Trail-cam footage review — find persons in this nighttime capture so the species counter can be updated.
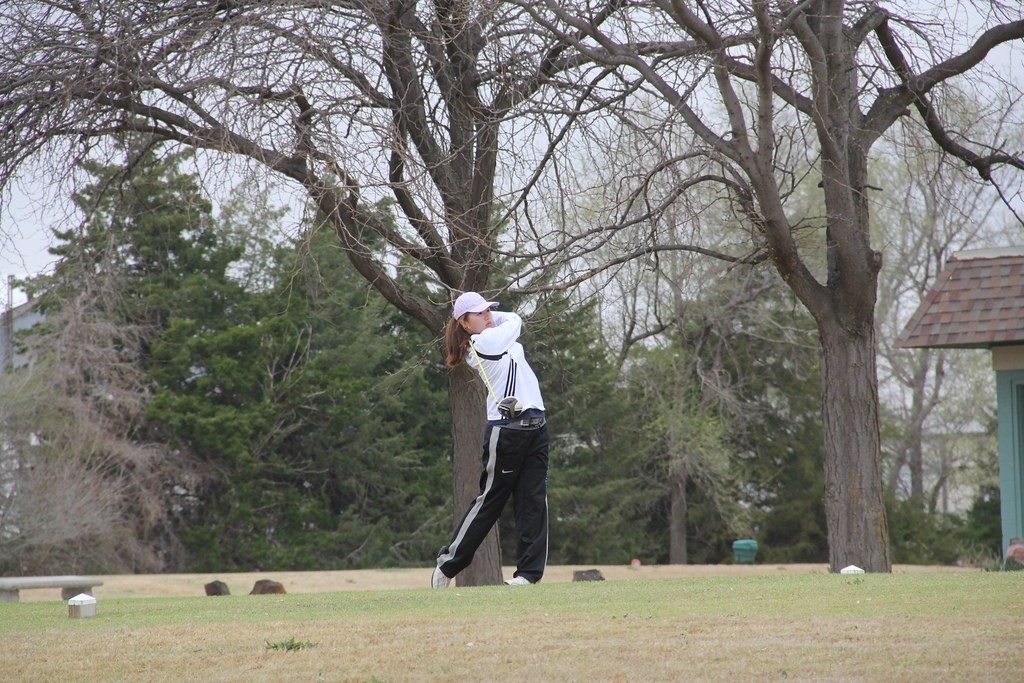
[431,292,550,586]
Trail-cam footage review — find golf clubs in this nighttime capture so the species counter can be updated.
[468,339,517,420]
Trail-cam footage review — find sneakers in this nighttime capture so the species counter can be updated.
[504,576,534,585]
[431,564,452,590]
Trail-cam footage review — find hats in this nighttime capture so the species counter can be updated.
[451,292,499,321]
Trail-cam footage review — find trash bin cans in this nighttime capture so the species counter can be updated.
[731,539,757,563]
